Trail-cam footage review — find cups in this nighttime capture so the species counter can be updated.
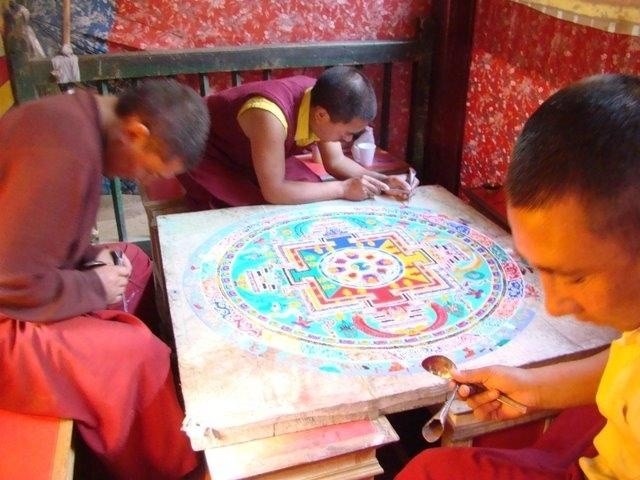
[357,143,377,168]
[311,143,322,163]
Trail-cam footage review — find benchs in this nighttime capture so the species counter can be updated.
[0,410,81,480]
[25,35,415,238]
[465,180,513,231]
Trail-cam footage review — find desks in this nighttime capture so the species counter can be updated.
[152,177,621,479]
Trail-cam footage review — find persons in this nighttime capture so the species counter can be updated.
[174,64,420,211]
[388,73,640,479]
[1,77,209,480]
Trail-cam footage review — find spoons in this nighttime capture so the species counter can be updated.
[422,355,528,414]
